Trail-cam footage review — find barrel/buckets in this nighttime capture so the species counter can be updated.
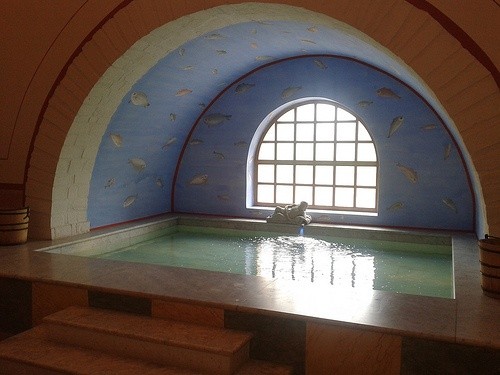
[0,206,31,245]
[478,234,500,293]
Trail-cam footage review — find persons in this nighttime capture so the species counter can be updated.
[270,200,309,219]
